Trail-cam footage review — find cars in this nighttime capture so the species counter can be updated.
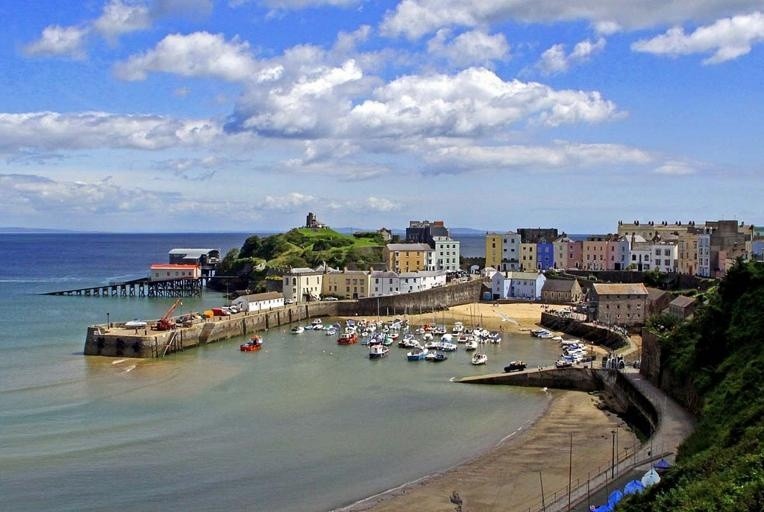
[325,297,338,301]
[504,361,527,373]
[211,305,239,316]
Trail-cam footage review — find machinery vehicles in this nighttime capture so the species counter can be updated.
[150,298,183,331]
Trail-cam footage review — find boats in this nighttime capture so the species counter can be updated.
[125,321,147,329]
[290,317,502,365]
[593,459,673,512]
[528,328,588,363]
[239,335,263,351]
